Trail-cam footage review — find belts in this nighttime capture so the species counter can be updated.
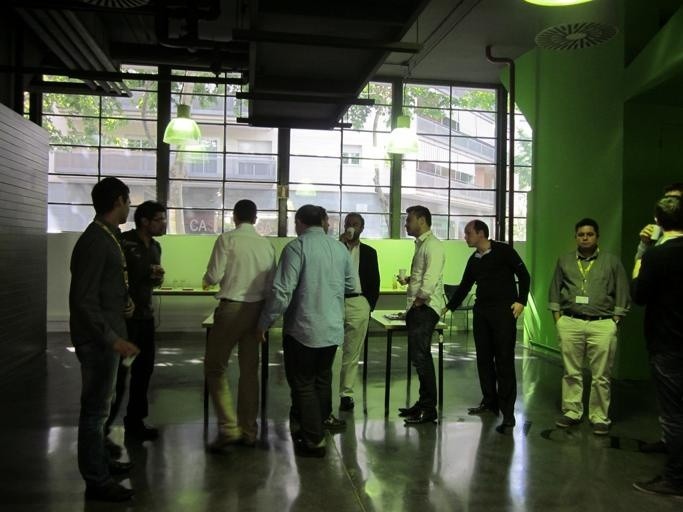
[344,293,363,298]
[563,309,615,323]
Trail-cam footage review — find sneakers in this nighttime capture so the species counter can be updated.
[638,440,664,456]
[556,416,585,429]
[591,422,611,436]
[632,475,682,499]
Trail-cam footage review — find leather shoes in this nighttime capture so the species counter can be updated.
[323,414,346,429]
[402,406,438,426]
[110,459,137,474]
[123,426,159,450]
[497,416,517,430]
[468,401,493,416]
[92,482,138,501]
[399,401,420,415]
[340,396,354,410]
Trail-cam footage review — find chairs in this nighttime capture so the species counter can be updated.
[441,285,476,336]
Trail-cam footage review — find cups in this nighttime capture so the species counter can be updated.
[345,227,355,239]
[200,276,216,292]
[398,268,408,282]
[151,265,161,278]
[649,224,661,241]
[121,346,141,368]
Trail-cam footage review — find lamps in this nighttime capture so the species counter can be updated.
[161,64,201,146]
[385,74,418,154]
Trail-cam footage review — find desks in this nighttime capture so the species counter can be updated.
[197,309,276,438]
[152,286,218,322]
[364,307,448,419]
[379,286,407,298]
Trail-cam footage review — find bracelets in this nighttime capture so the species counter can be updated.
[411,301,422,308]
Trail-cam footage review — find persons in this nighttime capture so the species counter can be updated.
[627,195,683,501]
[632,188,682,455]
[103,199,169,443]
[257,204,353,458]
[314,206,349,431]
[546,217,632,435]
[67,177,142,502]
[396,205,445,426]
[440,220,532,427]
[337,213,379,407]
[203,198,276,457]
